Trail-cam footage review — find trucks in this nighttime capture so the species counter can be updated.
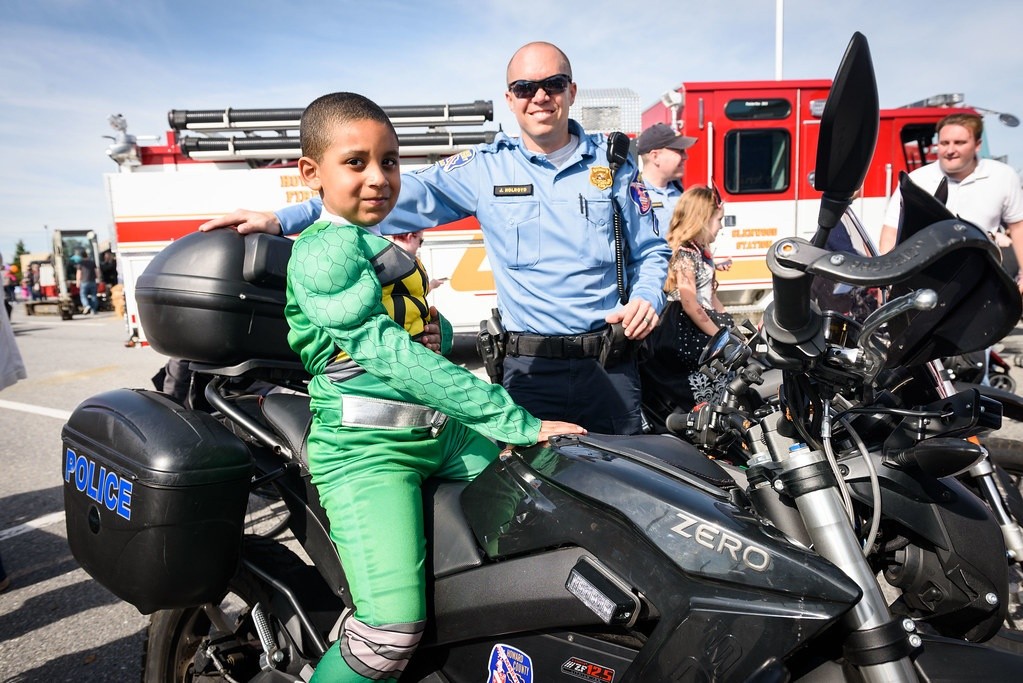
[102,73,1023,367]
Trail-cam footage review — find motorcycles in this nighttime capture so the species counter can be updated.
[59,29,1023,683]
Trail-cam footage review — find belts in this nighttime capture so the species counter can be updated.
[501,332,602,359]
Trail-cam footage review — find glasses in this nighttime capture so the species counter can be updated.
[508,74,572,98]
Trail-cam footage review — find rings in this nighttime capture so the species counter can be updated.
[645,318,650,323]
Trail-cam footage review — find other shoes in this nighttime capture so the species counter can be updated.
[84,306,92,314]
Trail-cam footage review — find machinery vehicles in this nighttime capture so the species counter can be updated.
[21,229,115,317]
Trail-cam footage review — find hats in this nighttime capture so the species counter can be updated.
[638,124,698,155]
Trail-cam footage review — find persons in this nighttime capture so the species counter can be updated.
[392,231,447,296]
[4,264,42,301]
[101,253,117,285]
[285,93,588,683]
[636,123,700,433]
[880,114,1023,393]
[76,251,97,316]
[658,185,732,371]
[201,42,670,432]
